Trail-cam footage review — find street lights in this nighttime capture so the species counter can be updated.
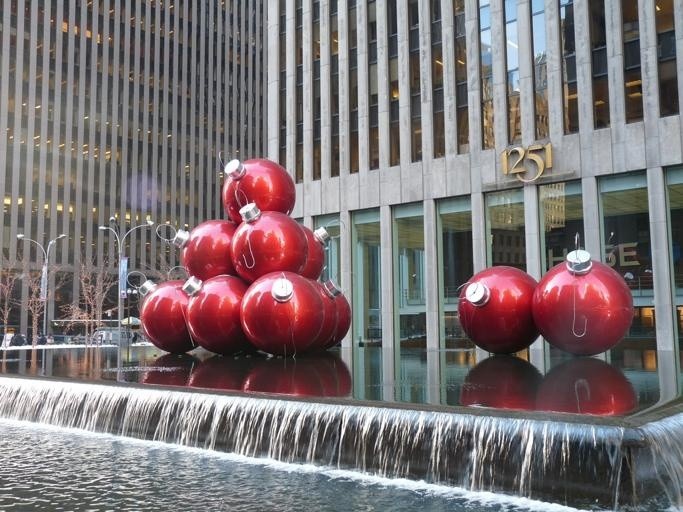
[98,216,154,346]
[16,233,67,336]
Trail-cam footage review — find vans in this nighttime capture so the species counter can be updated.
[94,331,141,345]
